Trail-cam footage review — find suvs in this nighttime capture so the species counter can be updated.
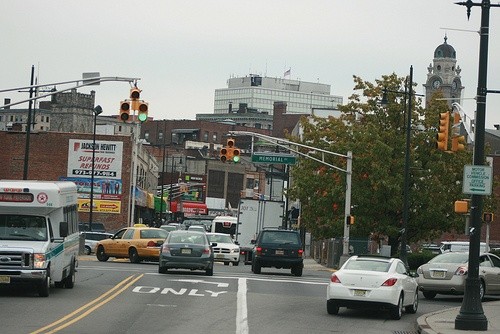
[205,232,241,266]
[80,232,114,255]
[250,227,305,277]
[418,241,490,253]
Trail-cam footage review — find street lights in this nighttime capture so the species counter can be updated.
[120,89,149,121]
[88,105,103,232]
[377,64,424,264]
[6,64,34,182]
[220,139,241,162]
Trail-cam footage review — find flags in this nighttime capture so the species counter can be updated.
[284,70,290,76]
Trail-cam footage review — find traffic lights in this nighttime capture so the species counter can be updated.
[452,112,465,153]
[435,109,450,152]
[347,216,354,224]
[483,213,492,223]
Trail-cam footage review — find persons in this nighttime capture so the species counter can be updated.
[115,181,119,194]
[110,180,115,194]
[106,179,110,194]
[102,180,106,193]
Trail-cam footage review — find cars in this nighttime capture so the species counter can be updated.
[417,251,500,300]
[326,254,419,319]
[95,223,169,263]
[159,230,218,276]
[160,215,237,240]
[79,222,105,233]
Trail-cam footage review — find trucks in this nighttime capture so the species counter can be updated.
[237,197,286,265]
[0,179,80,298]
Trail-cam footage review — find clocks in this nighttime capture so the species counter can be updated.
[433,80,441,88]
[452,81,457,89]
